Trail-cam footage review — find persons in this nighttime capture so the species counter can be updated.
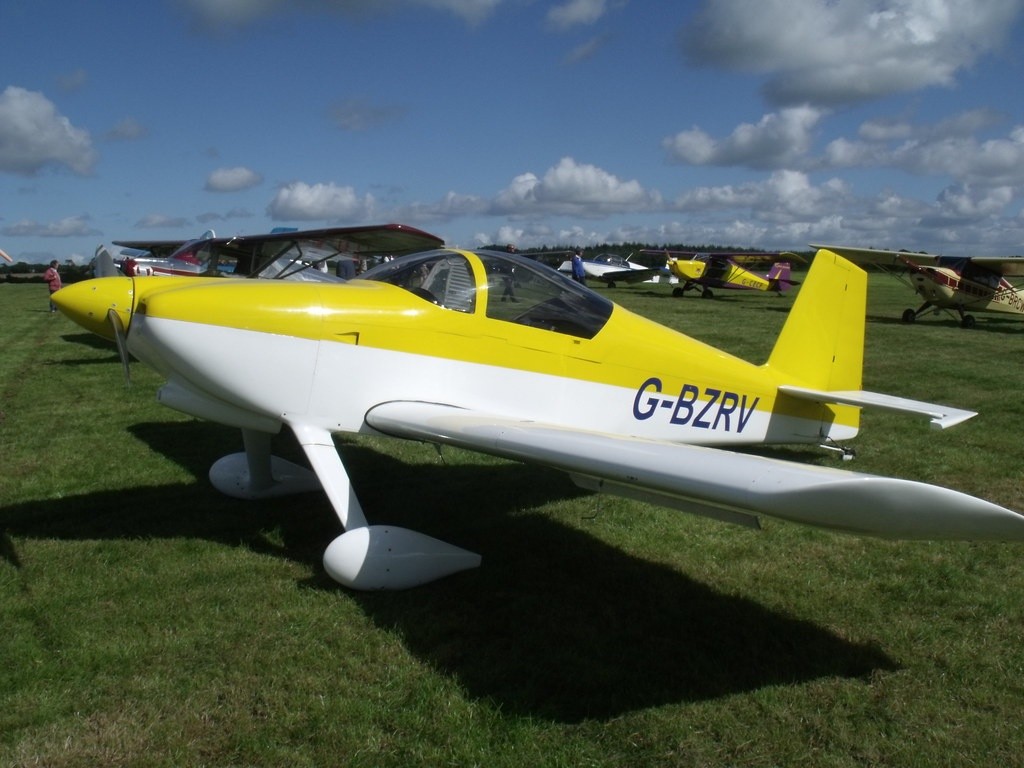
[196,255,238,268]
[571,248,587,288]
[499,243,521,303]
[125,256,130,264]
[43,259,63,313]
[119,258,128,275]
[126,259,136,276]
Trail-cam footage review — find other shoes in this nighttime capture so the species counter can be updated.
[501,299,506,302]
[512,300,520,303]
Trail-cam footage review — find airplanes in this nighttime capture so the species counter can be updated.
[638,244,808,301]
[555,251,678,289]
[38,248,1024,594]
[806,242,1023,330]
[108,223,447,280]
